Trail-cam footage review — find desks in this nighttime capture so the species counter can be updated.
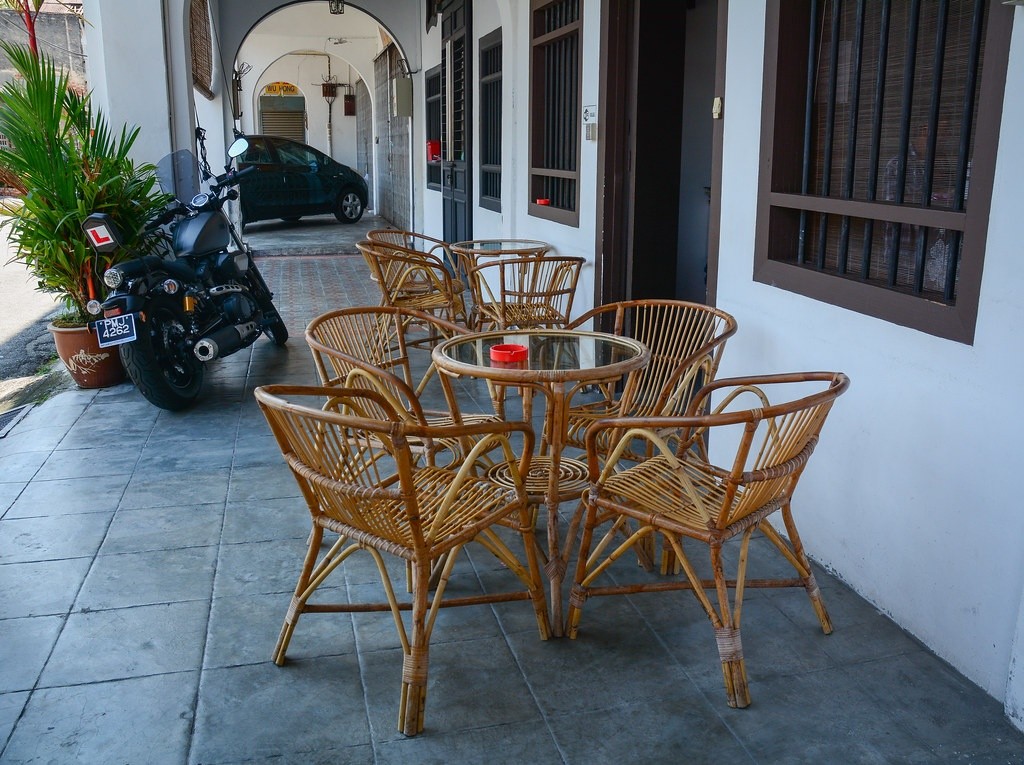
[450,239,551,377]
[431,328,649,638]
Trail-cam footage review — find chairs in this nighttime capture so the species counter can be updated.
[253,229,850,734]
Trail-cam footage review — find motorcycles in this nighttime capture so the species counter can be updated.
[85,137,289,413]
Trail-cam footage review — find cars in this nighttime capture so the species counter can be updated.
[235,134,369,228]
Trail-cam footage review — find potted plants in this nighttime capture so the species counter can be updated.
[0,0,175,388]
